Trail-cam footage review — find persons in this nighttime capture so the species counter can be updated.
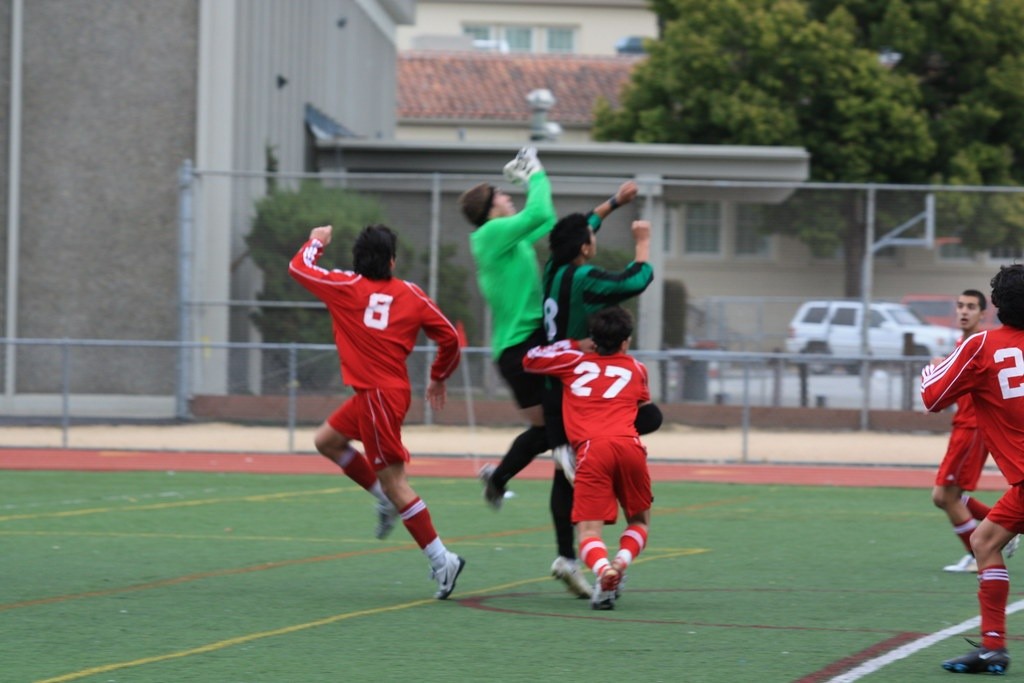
[458,145,562,508]
[288,223,466,601]
[523,305,652,612]
[921,264,1024,673]
[541,180,663,597]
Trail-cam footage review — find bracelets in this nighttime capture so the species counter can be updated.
[609,196,619,210]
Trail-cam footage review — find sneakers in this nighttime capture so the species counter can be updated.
[477,462,507,511]
[610,556,627,586]
[940,647,1010,675]
[549,555,595,600]
[1003,533,1020,559]
[943,553,981,573]
[430,550,466,600]
[590,563,620,611]
[373,500,399,540]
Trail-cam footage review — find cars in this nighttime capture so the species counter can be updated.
[786,300,964,375]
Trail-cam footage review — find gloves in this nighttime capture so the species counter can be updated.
[512,146,543,179]
[502,160,529,184]
